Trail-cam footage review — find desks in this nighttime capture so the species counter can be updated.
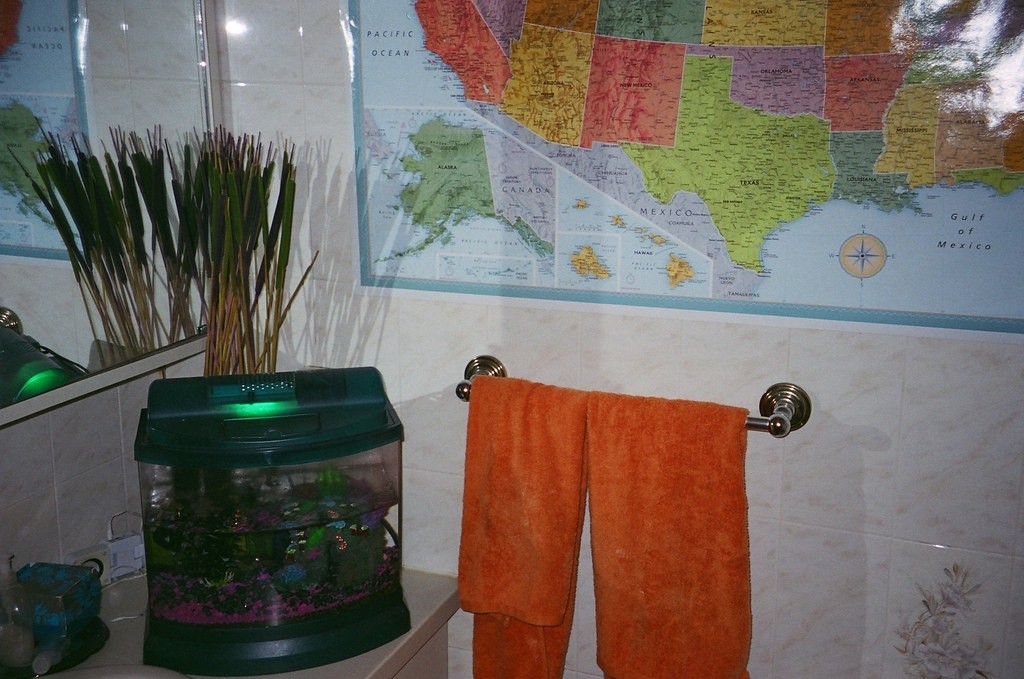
[34,563,462,679]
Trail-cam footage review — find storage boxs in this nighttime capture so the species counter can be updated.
[133,366,411,676]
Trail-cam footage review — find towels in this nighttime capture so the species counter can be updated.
[455,373,589,679]
[585,387,757,679]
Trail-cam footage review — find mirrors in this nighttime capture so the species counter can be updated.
[0,0,221,427]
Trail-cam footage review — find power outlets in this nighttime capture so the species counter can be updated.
[64,545,111,589]
[100,532,141,581]
